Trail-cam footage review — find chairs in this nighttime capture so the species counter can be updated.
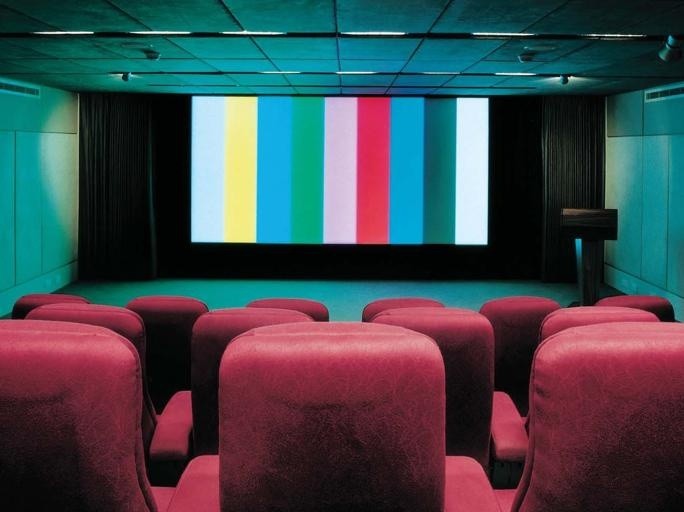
[21,301,163,462]
[478,294,563,415]
[595,294,678,320]
[445,320,682,509]
[359,296,447,323]
[122,296,210,394]
[2,317,177,510]
[163,317,446,512]
[487,304,664,492]
[369,306,497,471]
[244,296,334,327]
[145,307,317,484]
[9,292,87,316]
